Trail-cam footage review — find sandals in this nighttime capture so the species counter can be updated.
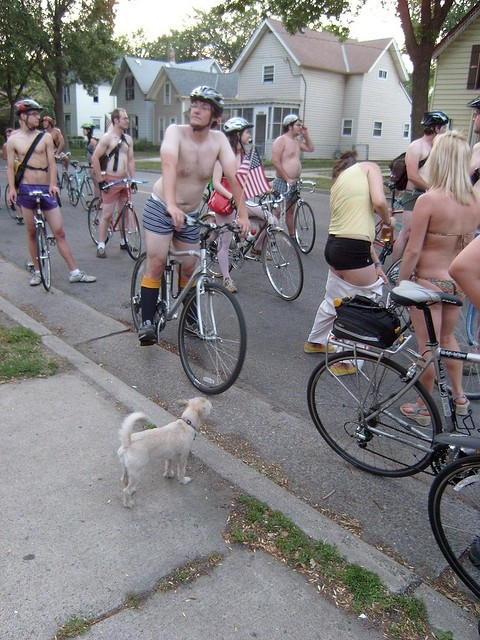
[400,404,432,426]
[452,395,470,414]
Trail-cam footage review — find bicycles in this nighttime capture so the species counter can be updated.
[200,190,304,301]
[277,179,316,254]
[4,182,23,220]
[129,210,248,395]
[67,159,100,211]
[88,177,150,260]
[54,150,75,204]
[373,180,405,264]
[436,297,480,400]
[425,436,477,598]
[11,189,56,292]
[306,279,480,479]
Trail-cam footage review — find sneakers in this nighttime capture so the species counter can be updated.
[251,249,274,260]
[70,272,96,282]
[97,247,106,260]
[304,342,337,354]
[330,363,358,376]
[184,319,212,340]
[30,272,42,285]
[120,243,139,252]
[138,320,158,346]
[223,278,237,294]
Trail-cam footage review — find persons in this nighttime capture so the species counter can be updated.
[91,108,139,259]
[212,116,279,294]
[0,127,13,158]
[466,94,480,206]
[269,114,315,248]
[392,111,449,270]
[448,235,480,316]
[396,128,480,428]
[139,86,250,345]
[304,151,396,376]
[7,100,98,285]
[42,115,69,182]
[81,123,100,211]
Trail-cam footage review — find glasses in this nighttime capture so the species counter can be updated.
[25,112,41,116]
[192,104,213,111]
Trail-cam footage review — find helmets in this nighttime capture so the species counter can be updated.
[80,122,95,129]
[42,116,53,123]
[13,99,43,115]
[465,95,480,108]
[190,86,226,111]
[223,117,253,132]
[420,111,449,125]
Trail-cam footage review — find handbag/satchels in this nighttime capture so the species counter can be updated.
[208,169,238,215]
[96,134,126,171]
[388,153,429,191]
[13,130,46,187]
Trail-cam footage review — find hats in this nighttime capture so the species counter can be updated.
[283,115,299,126]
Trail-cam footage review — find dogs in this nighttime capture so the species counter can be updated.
[118,396,214,509]
